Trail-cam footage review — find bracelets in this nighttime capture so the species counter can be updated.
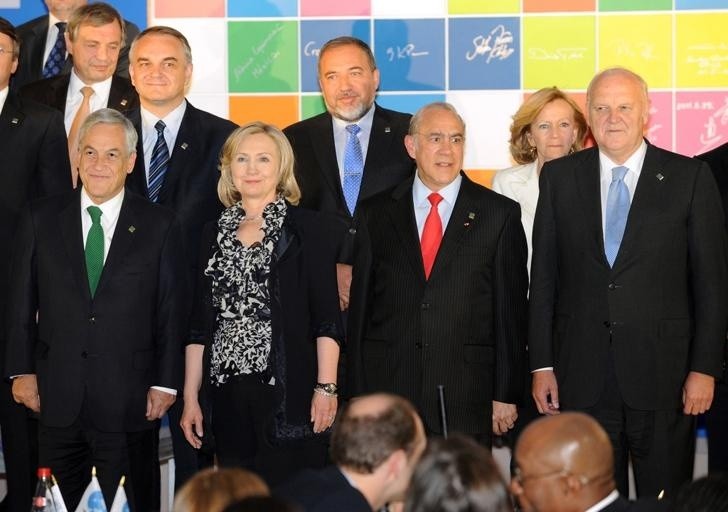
[314,382,340,398]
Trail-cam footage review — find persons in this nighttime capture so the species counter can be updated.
[271,393,429,511]
[19,3,140,192]
[118,26,241,512]
[506,408,681,512]
[526,66,723,503]
[1,16,74,512]
[690,144,728,483]
[490,84,591,304]
[8,107,187,512]
[285,36,419,402]
[170,466,271,511]
[179,122,345,490]
[346,102,530,460]
[401,431,515,512]
[7,1,143,92]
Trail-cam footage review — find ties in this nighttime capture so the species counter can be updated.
[84,206,104,300]
[342,124,364,218]
[420,192,444,281]
[42,22,66,80]
[147,120,169,203]
[67,87,95,190]
[604,166,631,270]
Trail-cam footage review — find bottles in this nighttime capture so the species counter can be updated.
[31,467,58,512]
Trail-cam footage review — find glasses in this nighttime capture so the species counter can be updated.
[0,48,13,56]
[515,466,570,485]
